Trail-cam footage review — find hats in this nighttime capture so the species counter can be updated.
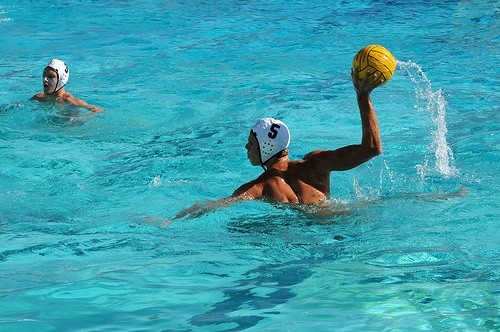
[44,59,69,95]
[250,116,290,163]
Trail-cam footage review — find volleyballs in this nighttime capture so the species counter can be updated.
[350,43,396,87]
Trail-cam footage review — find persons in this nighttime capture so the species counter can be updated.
[168,66,383,222]
[0,58,105,122]
